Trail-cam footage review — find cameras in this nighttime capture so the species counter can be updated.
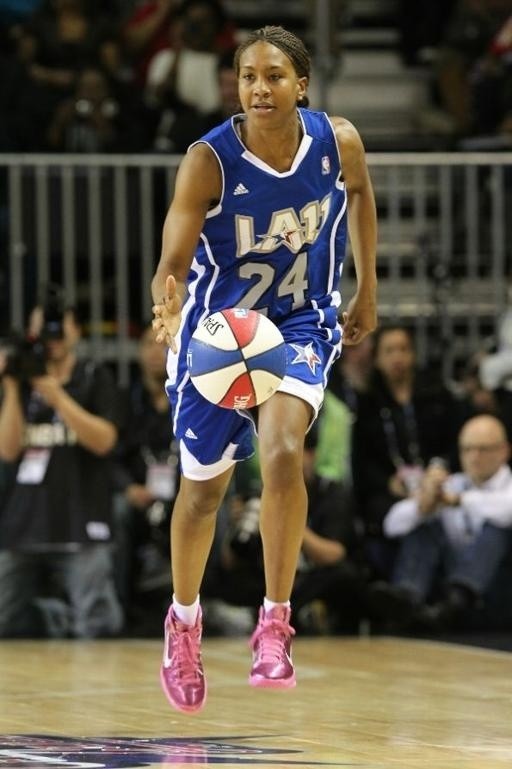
[6,335,47,381]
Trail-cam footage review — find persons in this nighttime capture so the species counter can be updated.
[0,1,512,340]
[151,26,377,710]
[1,304,512,639]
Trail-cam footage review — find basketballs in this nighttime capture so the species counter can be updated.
[188,309,287,410]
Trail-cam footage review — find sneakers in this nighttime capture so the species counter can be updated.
[248,603,297,690]
[158,603,207,715]
